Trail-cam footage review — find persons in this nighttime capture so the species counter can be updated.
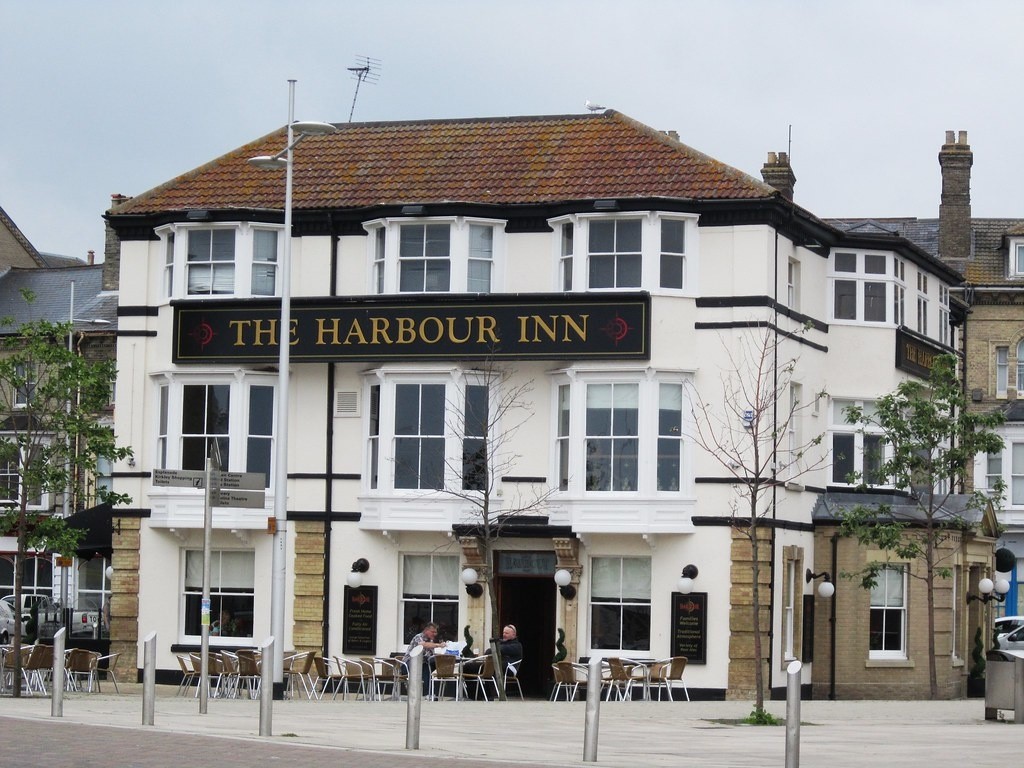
[103,596,112,636]
[400,622,447,701]
[209,609,234,636]
[482,624,523,701]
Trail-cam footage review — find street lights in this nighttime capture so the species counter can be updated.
[59,279,110,629]
[249,78,337,699]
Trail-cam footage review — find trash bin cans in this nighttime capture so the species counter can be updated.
[983,649,1024,724]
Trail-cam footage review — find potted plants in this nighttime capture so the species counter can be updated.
[967,627,985,697]
[543,628,573,701]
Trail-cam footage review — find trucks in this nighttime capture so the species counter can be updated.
[1,593,103,643]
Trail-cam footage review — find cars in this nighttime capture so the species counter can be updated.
[987,615,1024,661]
[0,599,30,657]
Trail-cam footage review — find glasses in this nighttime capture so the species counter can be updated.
[507,625,515,633]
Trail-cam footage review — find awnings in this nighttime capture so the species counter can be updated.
[63,501,111,561]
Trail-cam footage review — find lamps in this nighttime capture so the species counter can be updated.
[553,570,576,599]
[593,200,620,209]
[679,565,698,593]
[461,568,483,598]
[346,558,369,587]
[794,238,823,247]
[186,211,212,219]
[401,206,427,214]
[989,579,1010,602]
[806,568,834,597]
[967,578,993,605]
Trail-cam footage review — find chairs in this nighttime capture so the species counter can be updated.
[0,643,122,696]
[177,650,523,704]
[549,656,692,702]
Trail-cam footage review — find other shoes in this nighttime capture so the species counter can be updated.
[487,696,494,701]
[425,694,438,701]
